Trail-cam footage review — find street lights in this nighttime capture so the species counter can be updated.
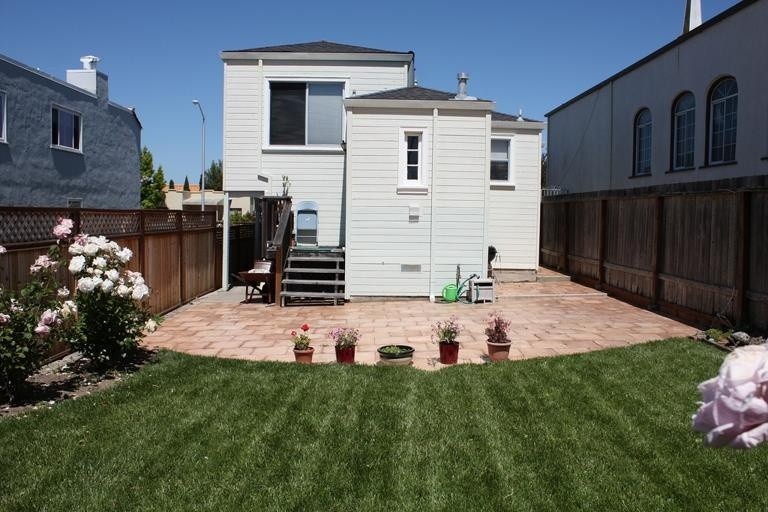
[192,99,207,220]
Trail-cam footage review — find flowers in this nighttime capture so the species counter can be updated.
[329,326,360,348]
[425,313,464,344]
[290,324,311,350]
[482,307,513,344]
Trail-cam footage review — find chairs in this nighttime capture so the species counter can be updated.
[240,258,274,303]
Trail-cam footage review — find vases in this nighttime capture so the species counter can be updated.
[292,346,314,365]
[487,340,512,364]
[437,342,460,365]
[335,346,355,366]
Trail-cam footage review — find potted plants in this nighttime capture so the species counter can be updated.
[376,342,416,368]
